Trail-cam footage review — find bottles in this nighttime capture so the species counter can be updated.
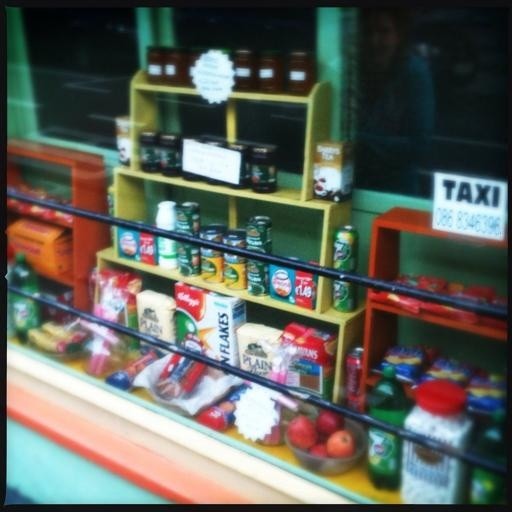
[138,128,281,194]
[145,44,316,96]
[400,378,475,506]
[467,408,508,504]
[365,365,411,491]
[8,252,41,343]
[155,200,180,269]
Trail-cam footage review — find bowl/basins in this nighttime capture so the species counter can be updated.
[284,411,366,476]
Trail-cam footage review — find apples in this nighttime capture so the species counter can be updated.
[286,409,357,458]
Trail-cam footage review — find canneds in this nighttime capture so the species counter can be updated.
[332,279,357,312]
[144,45,311,97]
[333,225,358,271]
[177,201,271,297]
[343,347,364,396]
[139,130,279,194]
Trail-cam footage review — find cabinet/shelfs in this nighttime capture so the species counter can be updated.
[8,136,110,322]
[93,71,369,409]
[356,205,512,417]
